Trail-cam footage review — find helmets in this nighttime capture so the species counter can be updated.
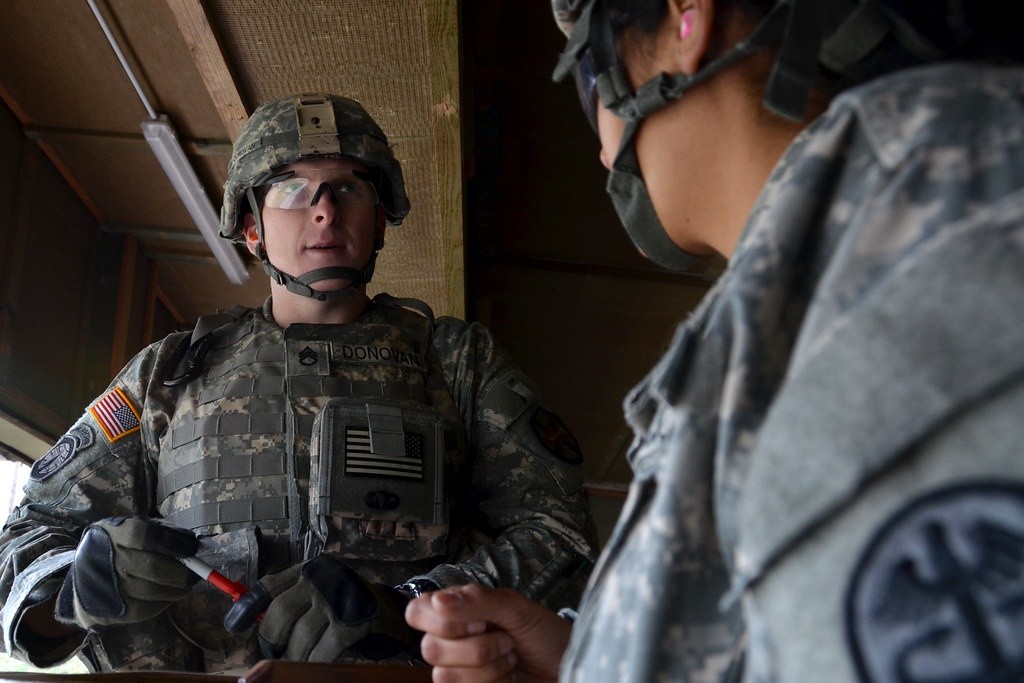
[220,94,411,237]
[551,0,952,275]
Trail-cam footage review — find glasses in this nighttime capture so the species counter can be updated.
[266,168,380,210]
[574,48,602,138]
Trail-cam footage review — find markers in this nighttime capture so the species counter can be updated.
[177,556,267,621]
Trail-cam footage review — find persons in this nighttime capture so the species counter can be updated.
[0,91,599,683]
[405,0,1024,683]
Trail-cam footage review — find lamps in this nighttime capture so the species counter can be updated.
[141,113,251,287]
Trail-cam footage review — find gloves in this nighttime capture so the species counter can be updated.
[55,514,202,628]
[224,558,408,661]
